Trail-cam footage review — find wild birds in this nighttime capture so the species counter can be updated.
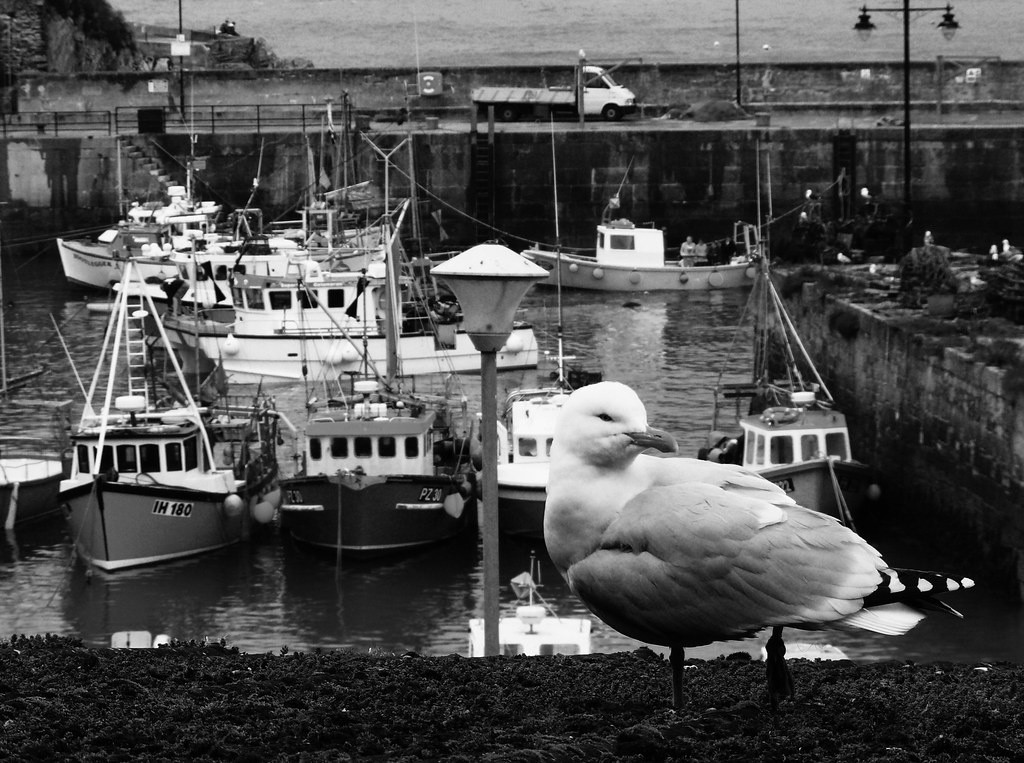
[989,239,1024,267]
[762,44,771,52]
[799,211,808,223]
[860,187,875,206]
[837,252,852,266]
[578,48,588,62]
[804,188,824,204]
[868,262,880,275]
[714,39,719,48]
[543,380,980,716]
[924,229,935,248]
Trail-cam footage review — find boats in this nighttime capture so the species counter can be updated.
[519,218,759,293]
[467,372,584,546]
[0,450,70,533]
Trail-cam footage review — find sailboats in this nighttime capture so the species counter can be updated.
[57,237,279,574]
[694,153,882,526]
[272,204,486,556]
[0,1,606,389]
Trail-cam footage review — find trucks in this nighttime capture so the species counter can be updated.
[469,66,636,123]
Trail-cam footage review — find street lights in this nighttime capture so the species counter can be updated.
[853,0,962,256]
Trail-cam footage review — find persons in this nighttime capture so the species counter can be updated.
[680,236,737,265]
[220,20,240,36]
